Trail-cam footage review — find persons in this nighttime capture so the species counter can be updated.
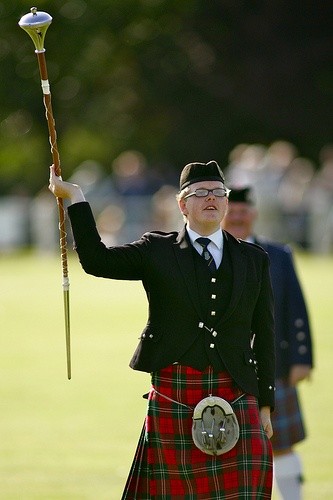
[222,184,316,500]
[48,161,277,500]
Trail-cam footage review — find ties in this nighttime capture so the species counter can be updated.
[195,238,216,274]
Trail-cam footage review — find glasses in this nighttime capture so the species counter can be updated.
[183,188,227,200]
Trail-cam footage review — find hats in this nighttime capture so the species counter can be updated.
[180,160,225,190]
[229,186,255,204]
